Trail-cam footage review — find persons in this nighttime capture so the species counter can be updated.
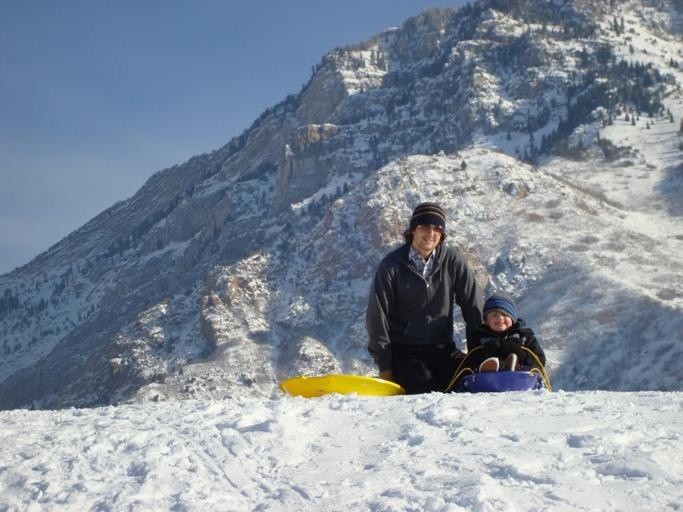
[478,293,545,373]
[365,202,486,395]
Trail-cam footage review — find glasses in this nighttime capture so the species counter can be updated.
[417,223,443,233]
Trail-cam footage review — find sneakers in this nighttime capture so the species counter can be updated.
[503,353,521,371]
[479,357,499,372]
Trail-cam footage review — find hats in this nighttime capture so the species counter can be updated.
[483,291,518,324]
[410,203,446,235]
